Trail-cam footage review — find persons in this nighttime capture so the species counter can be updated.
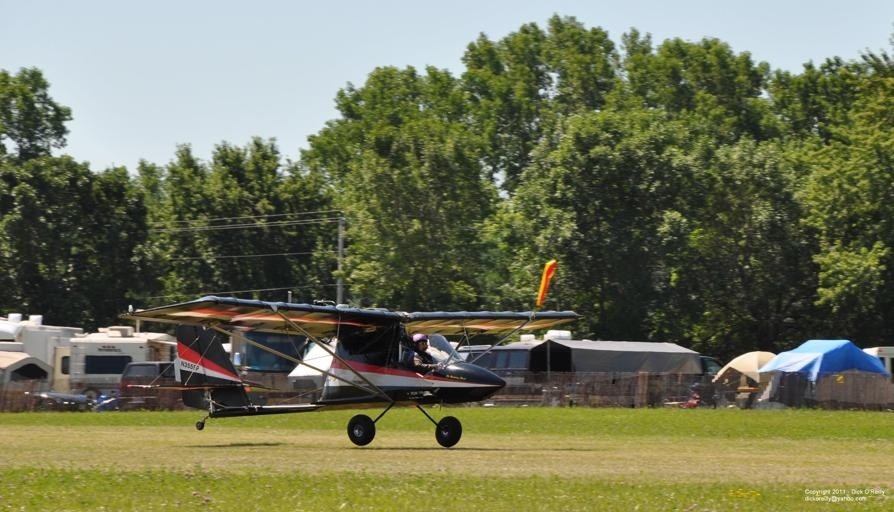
[410,334,444,369]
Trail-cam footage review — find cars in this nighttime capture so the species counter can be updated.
[243,380,282,406]
[32,390,118,412]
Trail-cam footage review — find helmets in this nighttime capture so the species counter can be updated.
[413,334,428,343]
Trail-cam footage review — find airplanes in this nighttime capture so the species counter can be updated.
[119,295,584,448]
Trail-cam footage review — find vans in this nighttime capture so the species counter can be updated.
[119,360,183,412]
